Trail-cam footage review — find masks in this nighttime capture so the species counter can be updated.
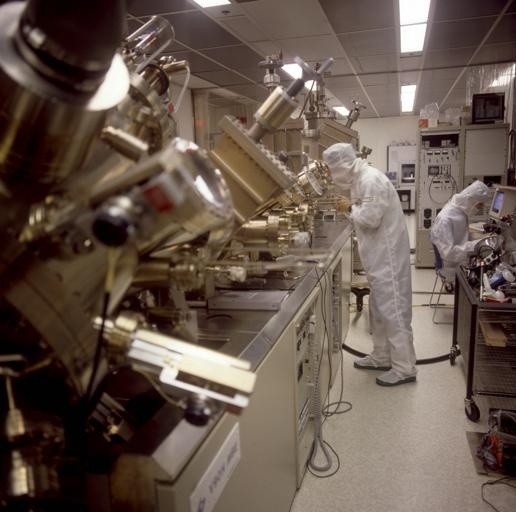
[471,206,480,216]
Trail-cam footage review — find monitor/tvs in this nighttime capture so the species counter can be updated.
[489,186,516,224]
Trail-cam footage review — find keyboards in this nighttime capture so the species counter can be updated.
[469,222,486,231]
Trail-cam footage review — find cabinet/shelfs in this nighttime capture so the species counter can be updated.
[450,262,516,422]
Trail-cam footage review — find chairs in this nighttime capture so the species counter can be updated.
[427,231,458,325]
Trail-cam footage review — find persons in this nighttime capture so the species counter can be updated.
[429,180,491,284]
[322,142,417,386]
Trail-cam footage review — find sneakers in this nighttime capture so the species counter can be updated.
[375,367,417,387]
[353,353,393,371]
